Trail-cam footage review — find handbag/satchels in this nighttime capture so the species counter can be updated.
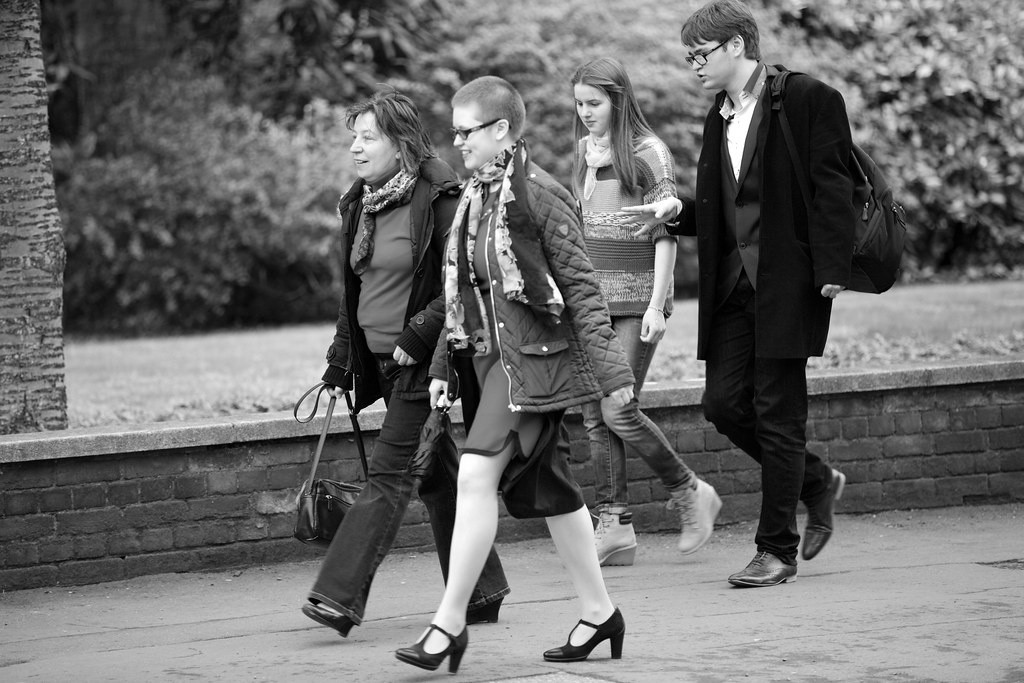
[293,478,364,552]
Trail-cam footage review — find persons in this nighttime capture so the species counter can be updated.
[614,0,857,586]
[570,59,723,566]
[302,86,512,638]
[394,76,637,674]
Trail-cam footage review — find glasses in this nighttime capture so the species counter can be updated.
[448,117,512,141]
[685,35,735,68]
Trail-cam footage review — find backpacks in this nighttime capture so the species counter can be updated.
[772,69,906,295]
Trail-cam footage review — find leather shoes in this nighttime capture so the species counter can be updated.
[800,469,846,561]
[727,551,798,587]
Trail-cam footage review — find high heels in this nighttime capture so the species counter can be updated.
[466,596,504,625]
[589,506,638,568]
[301,604,354,638]
[543,606,626,663]
[666,479,723,555]
[395,622,468,674]
